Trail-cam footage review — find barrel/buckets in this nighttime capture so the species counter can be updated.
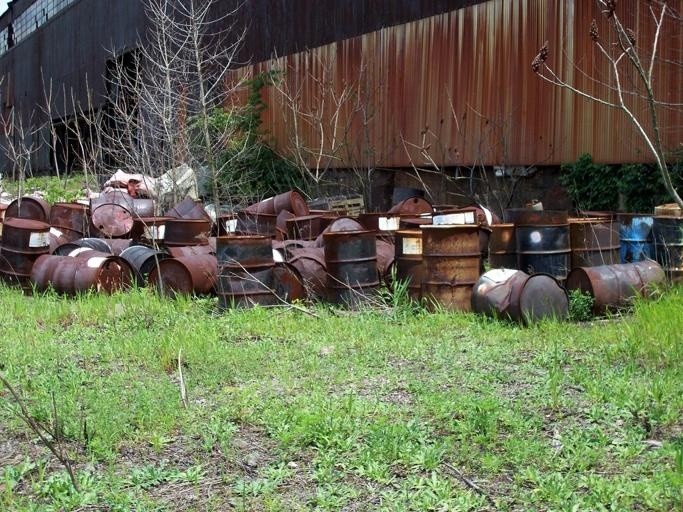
[0,167,683,328]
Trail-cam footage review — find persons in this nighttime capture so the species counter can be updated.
[127,179,150,199]
